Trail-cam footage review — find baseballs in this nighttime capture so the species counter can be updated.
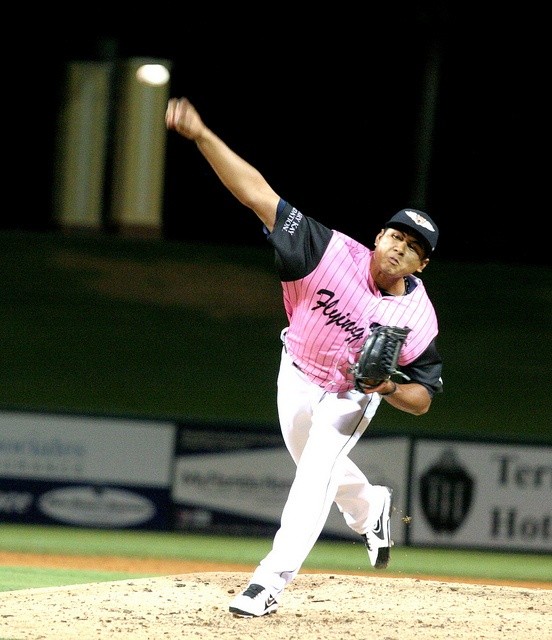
[136,64,169,86]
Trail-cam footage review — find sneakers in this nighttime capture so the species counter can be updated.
[228,584,278,617]
[360,485,394,569]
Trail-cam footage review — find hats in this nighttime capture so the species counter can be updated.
[385,208,440,252]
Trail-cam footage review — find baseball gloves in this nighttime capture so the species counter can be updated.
[348,322,411,394]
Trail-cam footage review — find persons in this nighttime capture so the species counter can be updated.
[165,97,444,617]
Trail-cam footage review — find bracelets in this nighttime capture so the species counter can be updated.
[379,382,397,396]
